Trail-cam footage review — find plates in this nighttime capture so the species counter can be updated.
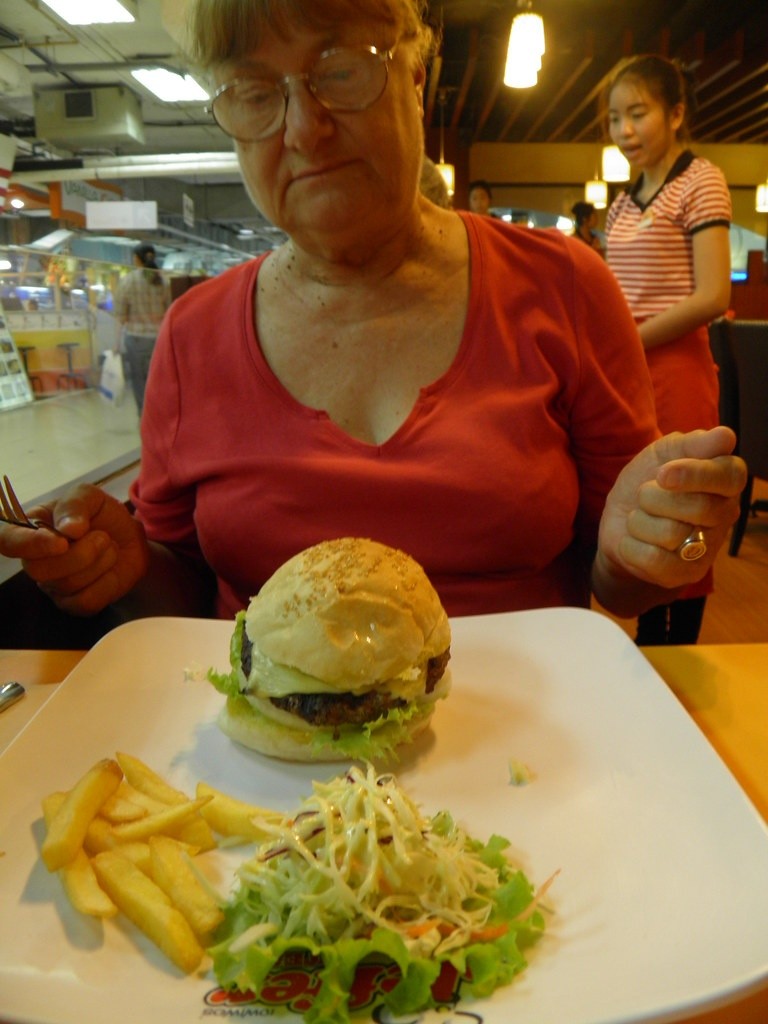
[1,608,768,1023]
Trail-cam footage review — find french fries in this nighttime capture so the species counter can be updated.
[40,752,292,975]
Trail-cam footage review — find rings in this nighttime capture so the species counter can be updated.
[671,526,707,560]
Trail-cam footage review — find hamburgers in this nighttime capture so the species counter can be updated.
[205,535,452,766]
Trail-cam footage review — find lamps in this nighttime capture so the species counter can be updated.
[584,142,635,211]
[432,86,456,197]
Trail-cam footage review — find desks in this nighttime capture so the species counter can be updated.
[1,648,768,1024]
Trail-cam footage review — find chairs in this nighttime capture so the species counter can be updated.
[709,314,767,556]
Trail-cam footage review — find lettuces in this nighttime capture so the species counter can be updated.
[209,809,545,1024]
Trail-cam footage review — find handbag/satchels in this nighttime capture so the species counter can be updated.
[97,351,126,406]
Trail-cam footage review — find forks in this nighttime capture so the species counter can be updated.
[2,471,79,545]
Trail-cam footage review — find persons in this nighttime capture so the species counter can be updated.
[585,54,732,646]
[1,0,746,626]
[566,200,604,259]
[466,179,500,220]
[110,239,171,423]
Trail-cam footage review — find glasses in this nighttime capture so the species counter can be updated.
[204,30,405,140]
